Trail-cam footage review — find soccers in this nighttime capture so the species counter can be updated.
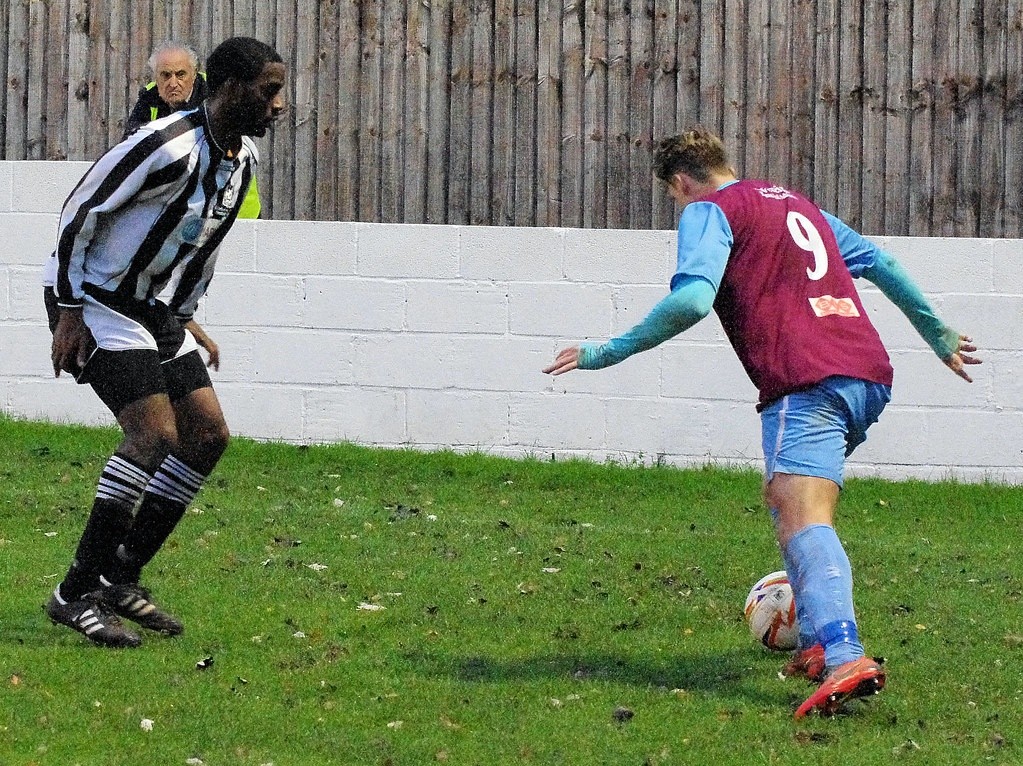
[743,570,800,650]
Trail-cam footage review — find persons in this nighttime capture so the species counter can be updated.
[121,42,262,220]
[44,38,289,648]
[542,129,984,717]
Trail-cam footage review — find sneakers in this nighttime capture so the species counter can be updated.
[795,656,887,719]
[787,642,828,683]
[46,580,143,649]
[98,574,184,636]
